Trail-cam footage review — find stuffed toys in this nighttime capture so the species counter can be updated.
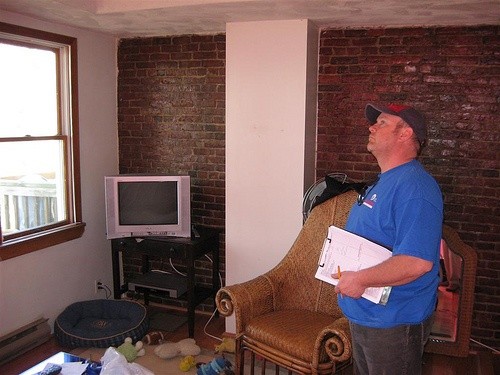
[214,337,236,356]
[114,338,146,362]
[154,337,201,359]
[179,355,195,371]
[196,354,237,375]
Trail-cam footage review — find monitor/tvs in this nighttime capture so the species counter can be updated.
[103,174,194,240]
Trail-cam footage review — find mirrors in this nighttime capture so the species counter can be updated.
[423,223,478,358]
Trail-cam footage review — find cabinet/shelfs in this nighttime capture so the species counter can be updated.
[104,228,225,340]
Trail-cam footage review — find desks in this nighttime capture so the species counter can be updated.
[16,352,101,375]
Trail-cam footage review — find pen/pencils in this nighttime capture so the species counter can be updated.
[338,266,343,300]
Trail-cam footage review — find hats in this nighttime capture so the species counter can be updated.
[365,102,429,149]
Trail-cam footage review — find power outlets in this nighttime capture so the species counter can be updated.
[94,278,104,295]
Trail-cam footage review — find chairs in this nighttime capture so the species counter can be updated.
[214,189,362,375]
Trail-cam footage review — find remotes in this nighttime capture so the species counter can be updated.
[193,230,200,237]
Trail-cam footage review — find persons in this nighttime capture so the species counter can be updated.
[330,103,445,375]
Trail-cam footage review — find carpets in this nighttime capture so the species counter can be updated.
[66,338,293,375]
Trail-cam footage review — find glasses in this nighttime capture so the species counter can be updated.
[356,173,380,206]
